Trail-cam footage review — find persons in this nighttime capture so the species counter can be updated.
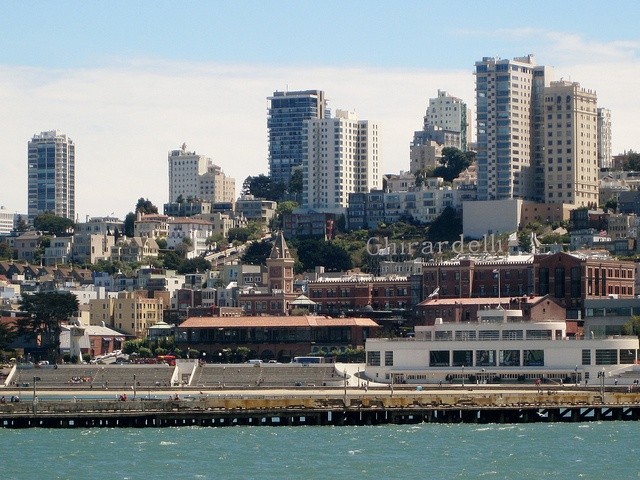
[0,395,20,403]
[67,376,91,384]
[169,395,181,401]
